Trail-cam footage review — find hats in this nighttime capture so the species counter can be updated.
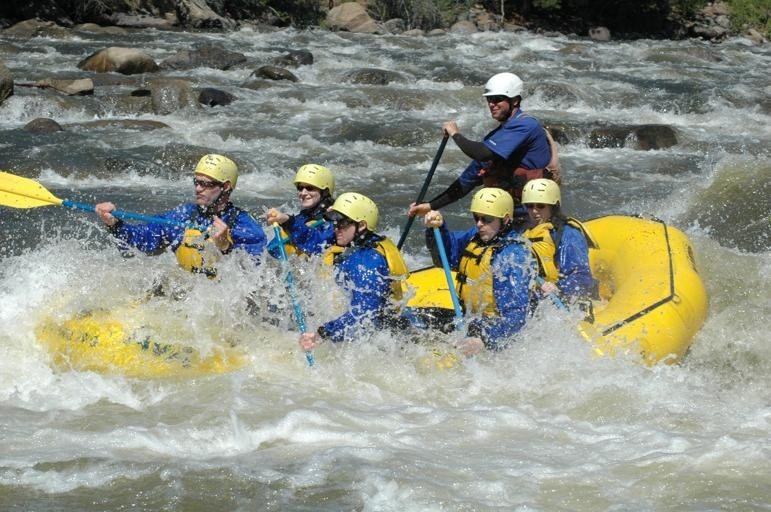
[322,211,347,222]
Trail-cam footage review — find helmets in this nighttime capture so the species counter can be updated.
[482,73,523,98]
[330,192,379,232]
[294,164,334,196]
[521,178,562,208]
[470,188,514,221]
[195,153,239,190]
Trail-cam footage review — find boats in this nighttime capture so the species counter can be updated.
[32,213,709,393]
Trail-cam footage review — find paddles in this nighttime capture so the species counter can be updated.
[0,169,214,235]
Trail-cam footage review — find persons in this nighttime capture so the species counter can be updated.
[519,177,601,325]
[266,191,410,354]
[96,153,268,280]
[424,187,538,359]
[408,72,561,233]
[266,165,334,260]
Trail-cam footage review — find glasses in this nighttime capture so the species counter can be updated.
[474,214,497,223]
[525,204,545,210]
[487,96,504,103]
[297,185,315,191]
[194,178,218,188]
[333,219,352,229]
